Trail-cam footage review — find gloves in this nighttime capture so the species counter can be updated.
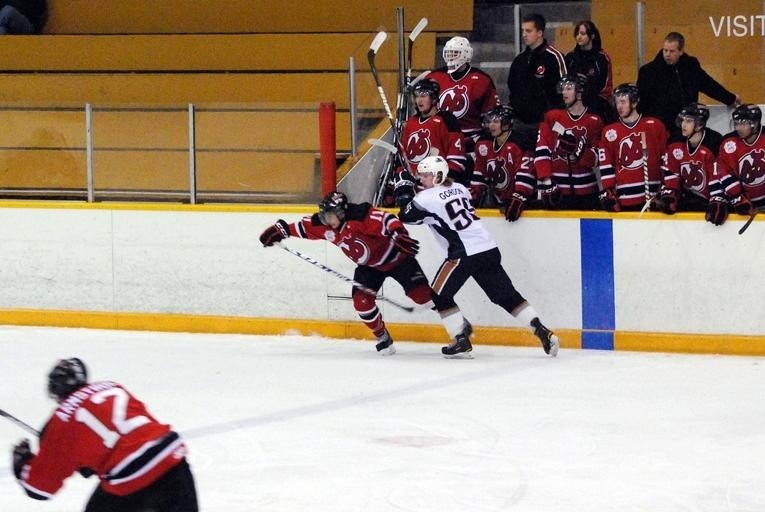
[14,441,31,478]
[500,192,528,222]
[388,230,419,257]
[468,184,487,208]
[556,134,584,160]
[392,169,422,199]
[729,192,760,215]
[259,220,290,247]
[705,193,730,226]
[650,184,679,215]
[541,183,564,210]
[597,188,621,212]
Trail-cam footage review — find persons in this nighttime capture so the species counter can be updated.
[717,102,765,215]
[634,33,742,153]
[12,356,198,511]
[393,155,554,356]
[394,78,475,208]
[656,102,728,225]
[534,73,604,210]
[599,82,668,213]
[507,13,568,147]
[469,105,536,222]
[402,35,500,166]
[564,22,616,123]
[260,190,472,351]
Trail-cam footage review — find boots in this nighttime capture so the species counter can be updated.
[375,328,393,351]
[442,328,472,354]
[531,317,553,354]
[462,315,472,336]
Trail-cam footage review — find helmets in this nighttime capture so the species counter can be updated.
[443,36,474,74]
[417,156,449,177]
[730,104,762,131]
[49,358,86,401]
[556,72,587,94]
[609,83,639,107]
[318,191,347,226]
[412,78,440,100]
[481,105,513,132]
[675,103,710,128]
